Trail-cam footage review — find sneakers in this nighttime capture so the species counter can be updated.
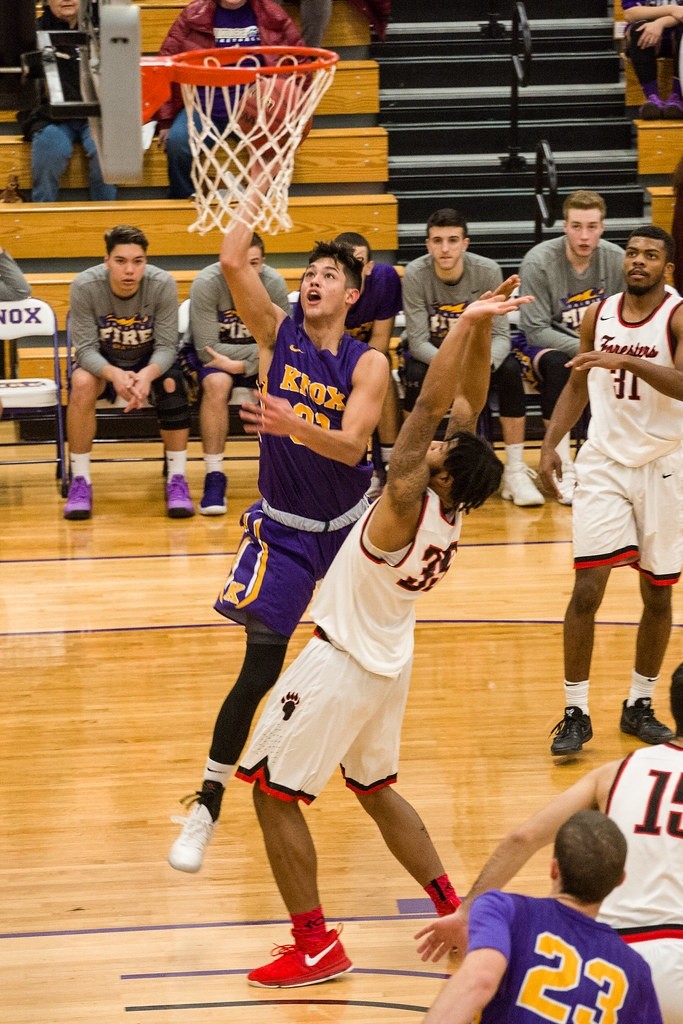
[620,697,674,743]
[63,476,92,520]
[502,462,545,506]
[167,804,218,873]
[246,922,353,989]
[548,706,593,755]
[551,462,575,504]
[165,474,194,517]
[663,93,683,119]
[198,472,227,515]
[639,94,661,119]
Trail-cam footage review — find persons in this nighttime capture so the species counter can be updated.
[155,0,313,201]
[534,227,683,759]
[233,274,534,991]
[335,232,399,498]
[400,211,547,506]
[420,809,666,1024]
[275,0,332,60]
[621,0,683,120]
[21,0,118,203]
[414,662,683,1024]
[516,191,632,505]
[170,154,388,876]
[119,234,291,543]
[0,245,31,302]
[62,227,196,518]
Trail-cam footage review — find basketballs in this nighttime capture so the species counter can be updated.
[236,78,314,159]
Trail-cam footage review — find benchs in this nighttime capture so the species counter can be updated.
[0,0,400,411]
[613,0,683,290]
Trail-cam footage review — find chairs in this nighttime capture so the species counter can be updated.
[0,297,68,498]
